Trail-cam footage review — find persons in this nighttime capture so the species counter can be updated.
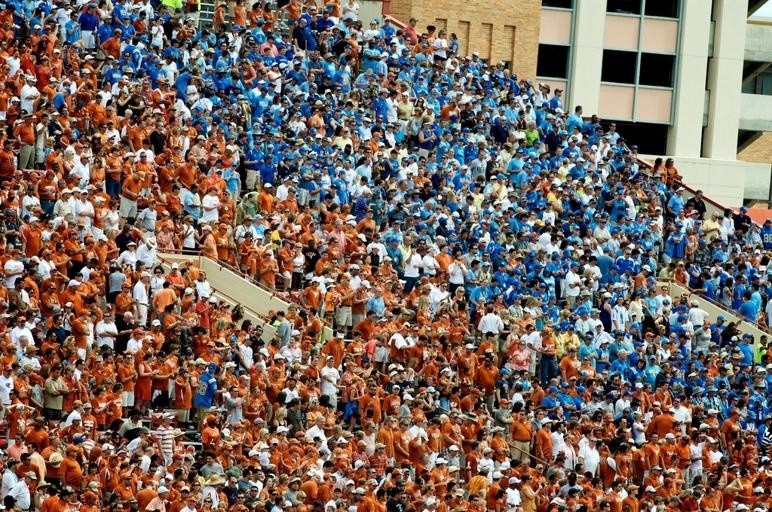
[0,0,770,512]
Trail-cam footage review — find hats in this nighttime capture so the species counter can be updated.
[2,5,771,510]
